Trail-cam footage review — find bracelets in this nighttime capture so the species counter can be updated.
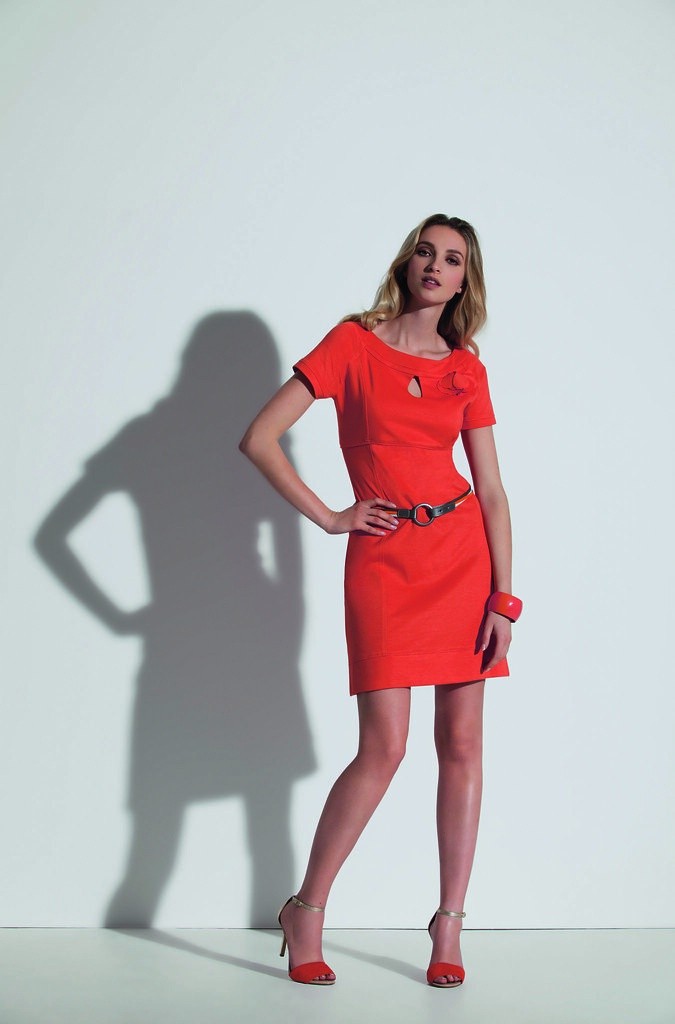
[486,592,523,622]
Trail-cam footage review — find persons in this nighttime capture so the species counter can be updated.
[239,214,513,987]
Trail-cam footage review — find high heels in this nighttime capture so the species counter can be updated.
[278,895,336,986]
[426,908,466,988]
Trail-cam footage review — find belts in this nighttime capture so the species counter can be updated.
[384,487,474,526]
